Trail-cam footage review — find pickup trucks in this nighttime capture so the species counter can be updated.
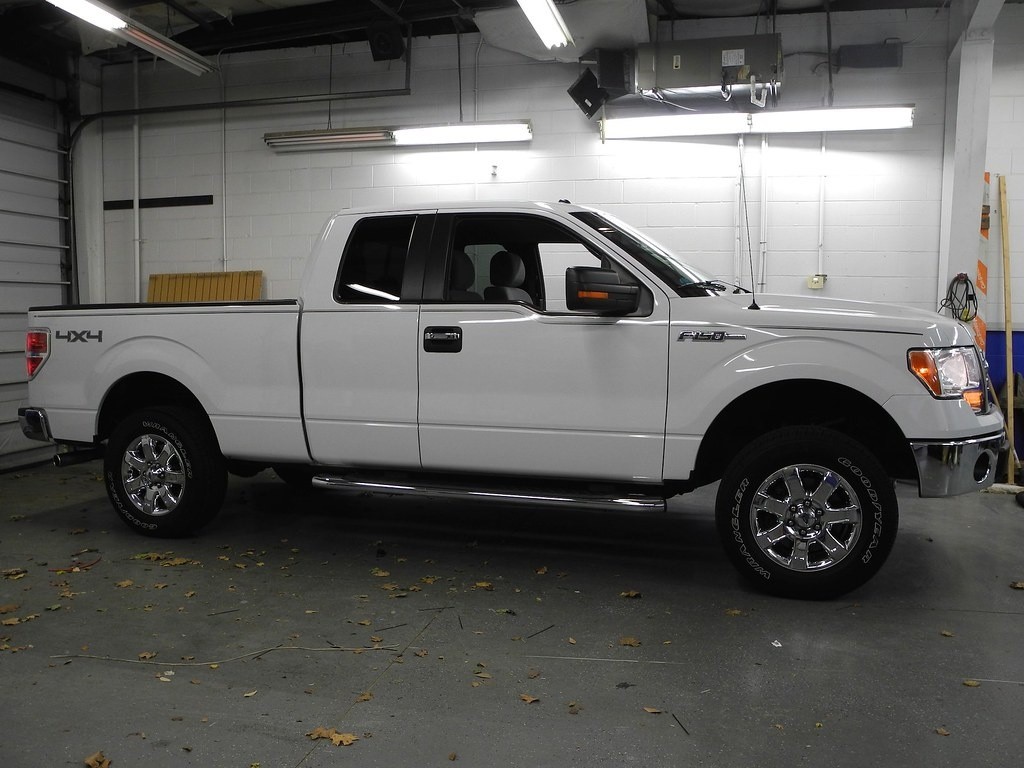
[19,193,1010,599]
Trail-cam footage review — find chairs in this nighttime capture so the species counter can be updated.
[484,249,534,308]
[448,251,485,305]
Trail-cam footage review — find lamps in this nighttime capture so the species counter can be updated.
[263,118,534,154]
[516,1,578,52]
[45,0,227,78]
[599,101,918,144]
